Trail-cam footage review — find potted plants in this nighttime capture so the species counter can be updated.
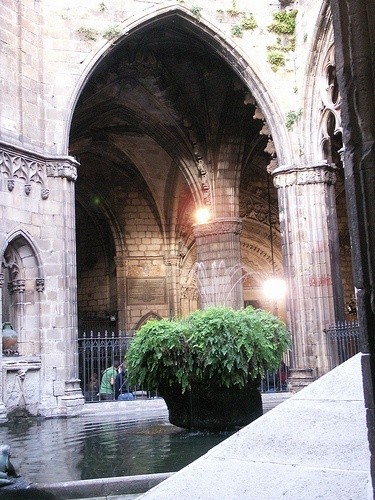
[115,304,293,432]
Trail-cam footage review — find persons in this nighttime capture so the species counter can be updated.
[87,360,134,402]
[264,362,288,391]
